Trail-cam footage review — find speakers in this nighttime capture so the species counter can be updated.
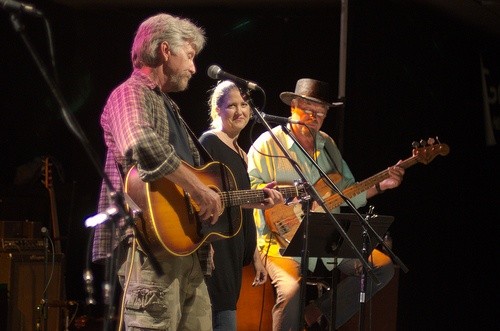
[326,263,399,331]
[9,254,62,330]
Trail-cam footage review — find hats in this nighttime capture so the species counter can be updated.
[279,78,343,107]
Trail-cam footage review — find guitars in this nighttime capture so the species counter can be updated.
[40,154,62,254]
[264,138,450,248]
[123,159,311,260]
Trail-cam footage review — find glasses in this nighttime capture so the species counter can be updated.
[300,107,327,118]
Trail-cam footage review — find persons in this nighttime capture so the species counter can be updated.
[91,12,283,331]
[198,80,268,331]
[247,79,406,331]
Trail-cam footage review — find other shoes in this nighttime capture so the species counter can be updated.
[303,307,322,331]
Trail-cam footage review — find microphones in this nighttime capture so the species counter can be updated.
[207,64,257,91]
[255,111,304,126]
[0,0,43,17]
[48,299,74,306]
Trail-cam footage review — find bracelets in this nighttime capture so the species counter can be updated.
[375,183,381,194]
